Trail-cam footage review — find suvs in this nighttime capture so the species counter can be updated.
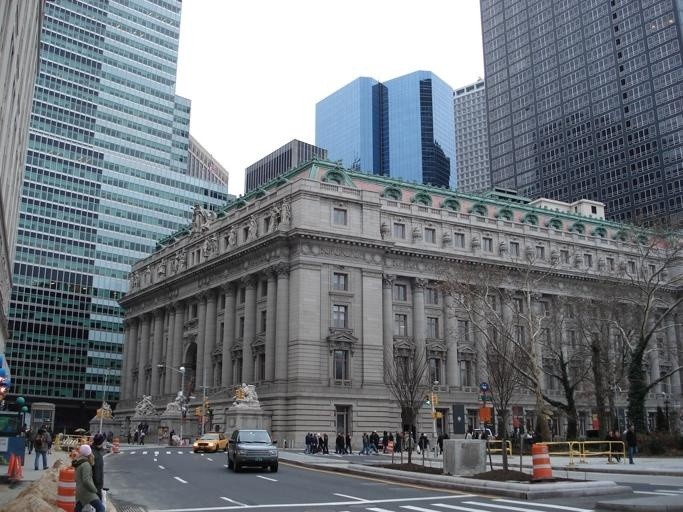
[227,429,279,475]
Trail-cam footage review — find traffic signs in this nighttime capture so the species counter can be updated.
[479,394,496,401]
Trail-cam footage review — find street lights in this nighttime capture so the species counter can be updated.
[22,406,29,438]
[16,396,25,437]
[432,381,440,457]
[664,399,671,431]
[99,367,111,435]
[609,371,623,433]
[199,386,209,435]
[156,364,185,447]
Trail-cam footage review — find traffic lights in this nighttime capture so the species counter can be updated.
[236,388,245,400]
[195,407,200,416]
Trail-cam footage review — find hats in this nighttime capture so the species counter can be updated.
[93,433,106,445]
[79,444,91,457]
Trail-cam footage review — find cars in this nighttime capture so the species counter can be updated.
[193,431,229,454]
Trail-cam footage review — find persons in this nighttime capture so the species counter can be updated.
[137,394,152,413]
[71,445,105,511]
[25,424,55,454]
[603,423,640,464]
[33,424,51,470]
[125,430,146,445]
[128,197,294,292]
[88,433,105,500]
[303,428,536,455]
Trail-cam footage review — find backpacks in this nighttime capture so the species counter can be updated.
[35,433,45,449]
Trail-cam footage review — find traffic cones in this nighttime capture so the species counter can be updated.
[6,453,24,479]
